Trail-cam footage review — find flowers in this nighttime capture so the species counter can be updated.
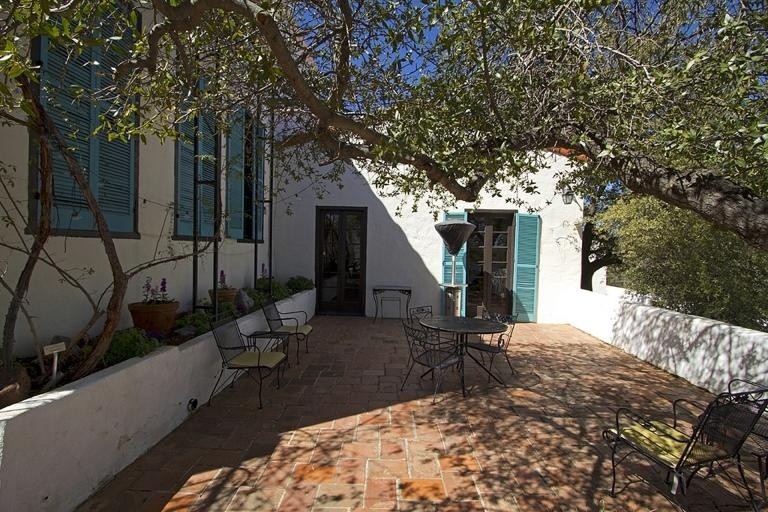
[141,276,169,301]
[217,271,228,288]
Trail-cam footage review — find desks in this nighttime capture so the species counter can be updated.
[372,286,412,321]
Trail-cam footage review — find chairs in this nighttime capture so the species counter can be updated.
[602,378,768,511]
[400,304,520,404]
[208,296,314,410]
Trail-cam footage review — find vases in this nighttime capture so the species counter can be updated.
[128,302,178,338]
[207,288,236,303]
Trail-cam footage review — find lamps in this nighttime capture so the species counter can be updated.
[561,187,584,211]
